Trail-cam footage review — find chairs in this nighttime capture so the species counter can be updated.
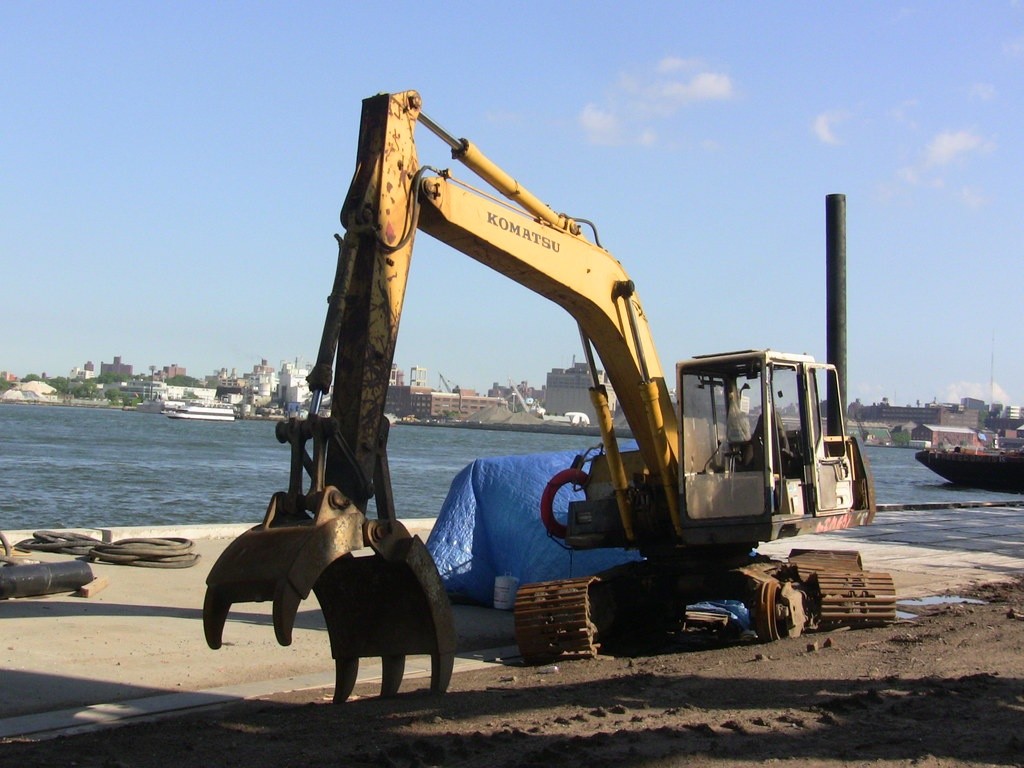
[743,410,798,473]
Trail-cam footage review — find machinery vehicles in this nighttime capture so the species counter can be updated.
[210,87,899,706]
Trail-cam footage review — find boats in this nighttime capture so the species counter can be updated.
[913,446,1024,489]
[161,399,237,423]
